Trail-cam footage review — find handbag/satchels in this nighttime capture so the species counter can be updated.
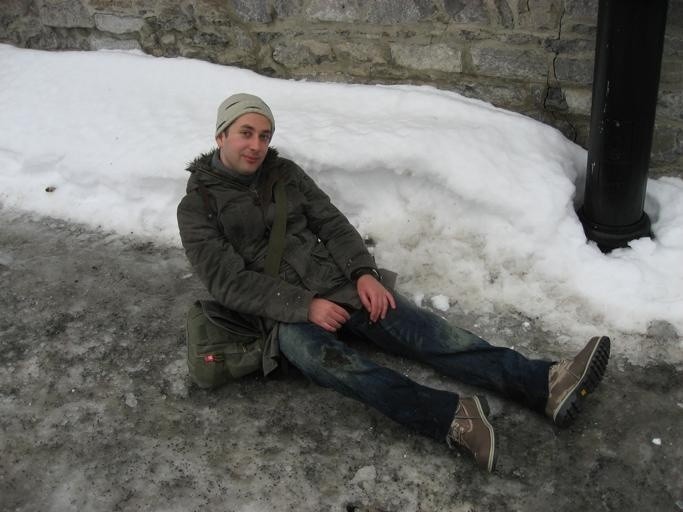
[186,299,267,392]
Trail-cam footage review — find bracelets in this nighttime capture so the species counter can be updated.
[352,267,383,285]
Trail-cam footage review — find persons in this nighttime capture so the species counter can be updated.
[177,94,612,473]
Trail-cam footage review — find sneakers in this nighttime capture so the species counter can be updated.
[445,394,499,474]
[544,336,610,428]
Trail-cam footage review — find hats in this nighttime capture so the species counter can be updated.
[216,93,275,137]
